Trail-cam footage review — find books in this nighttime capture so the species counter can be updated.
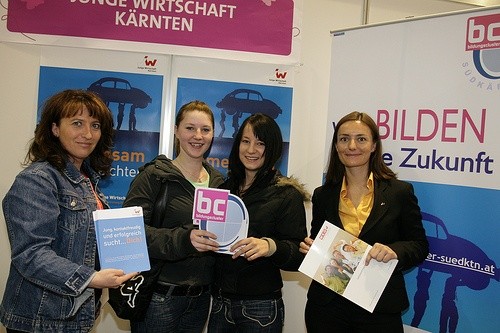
[92,206,151,279]
[298,220,398,313]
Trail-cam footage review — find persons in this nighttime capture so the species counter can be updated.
[0,88,140,333]
[320,240,368,296]
[298,111,430,333]
[207,113,308,333]
[121,99,227,333]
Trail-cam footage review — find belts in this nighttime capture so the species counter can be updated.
[153,281,208,298]
[221,289,282,301]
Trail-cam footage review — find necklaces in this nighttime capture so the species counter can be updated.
[175,158,206,183]
